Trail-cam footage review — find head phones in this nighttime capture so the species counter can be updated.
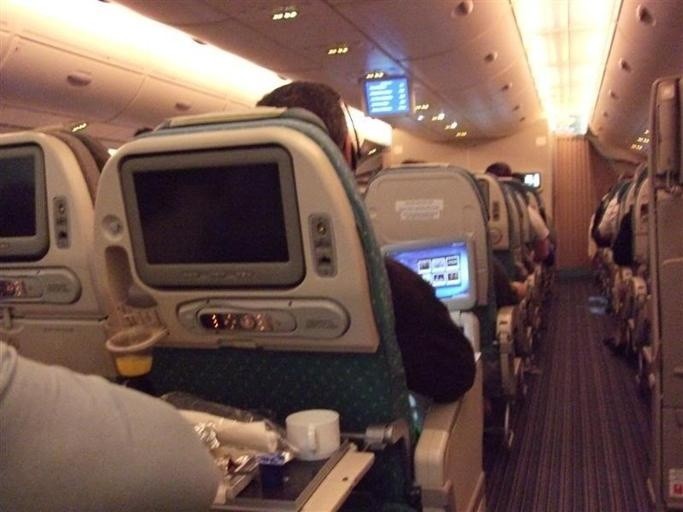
[348,139,363,168]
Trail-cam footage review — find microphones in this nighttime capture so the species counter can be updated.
[344,100,364,155]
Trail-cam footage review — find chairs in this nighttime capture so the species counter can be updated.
[1,103,554,512]
[591,76,682,512]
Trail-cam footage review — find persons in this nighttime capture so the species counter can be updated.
[585,158,660,280]
[0,340,222,511]
[468,162,550,303]
[252,80,475,402]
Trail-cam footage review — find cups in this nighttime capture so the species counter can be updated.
[97,312,155,377]
[283,408,340,464]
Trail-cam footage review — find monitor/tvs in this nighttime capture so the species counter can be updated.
[382,237,476,311]
[522,173,541,188]
[0,142,49,260]
[361,73,415,116]
[117,144,306,288]
